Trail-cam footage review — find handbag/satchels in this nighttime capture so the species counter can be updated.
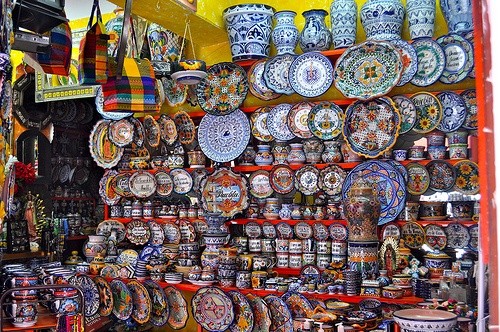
[37,0,161,114]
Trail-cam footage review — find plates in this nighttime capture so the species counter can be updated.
[68,277,315,332]
[88,33,476,218]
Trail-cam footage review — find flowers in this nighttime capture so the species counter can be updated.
[4,155,36,194]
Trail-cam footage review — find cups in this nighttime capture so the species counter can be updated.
[84,130,479,295]
[0,259,85,331]
[296,300,478,332]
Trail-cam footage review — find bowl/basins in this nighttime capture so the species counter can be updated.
[391,308,457,332]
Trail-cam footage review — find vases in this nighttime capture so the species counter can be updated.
[399,200,475,221]
[105,8,187,62]
[85,235,106,257]
[187,130,469,168]
[222,0,474,62]
[263,198,346,221]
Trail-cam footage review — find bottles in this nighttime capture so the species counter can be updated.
[107,9,182,62]
[272,0,474,55]
[344,187,380,241]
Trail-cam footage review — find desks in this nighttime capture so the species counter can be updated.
[91,275,426,332]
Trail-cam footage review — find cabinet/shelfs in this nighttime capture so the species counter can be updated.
[104,0,499,332]
